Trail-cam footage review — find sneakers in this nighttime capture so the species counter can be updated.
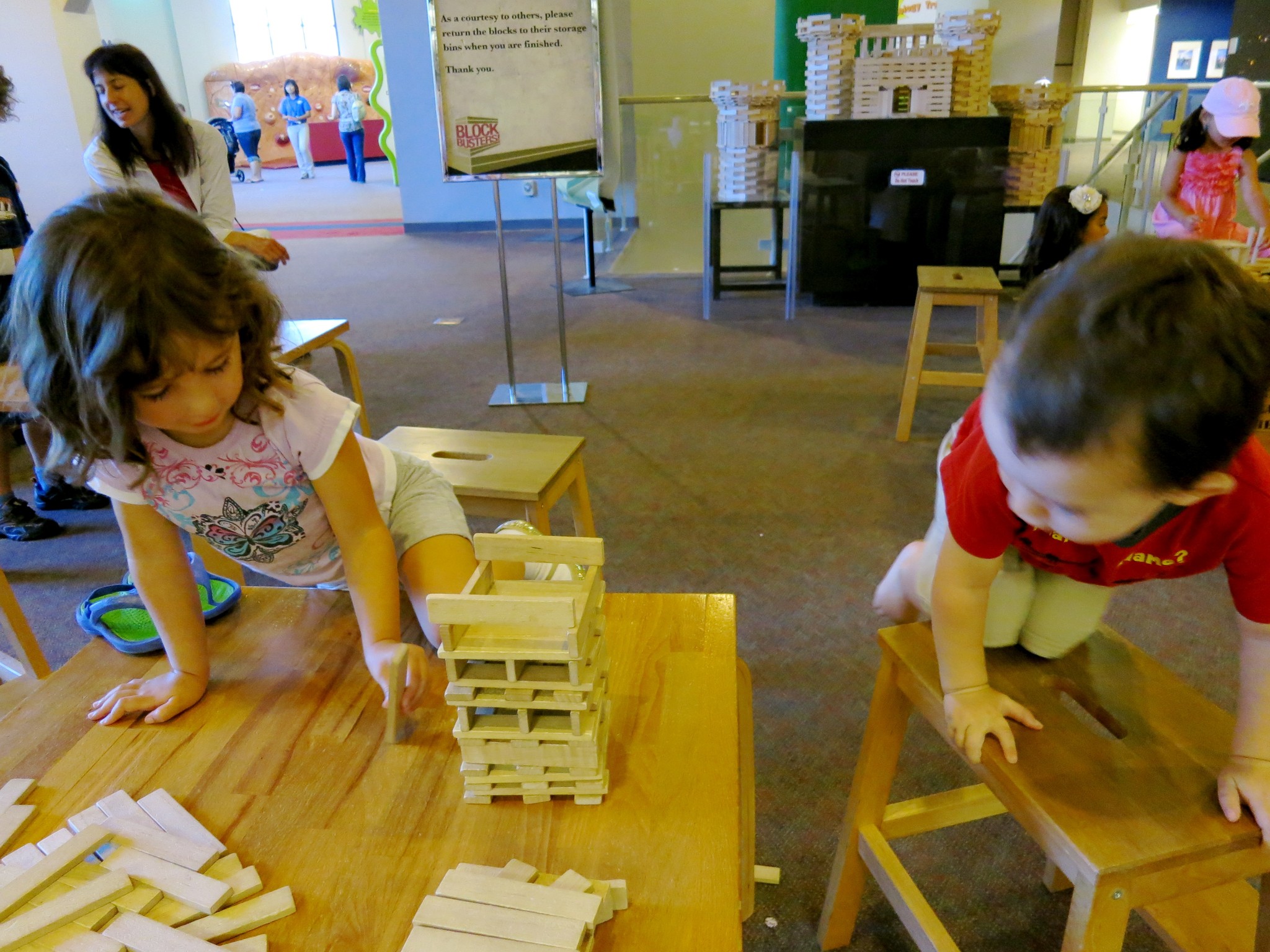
[0,492,59,542]
[30,477,111,511]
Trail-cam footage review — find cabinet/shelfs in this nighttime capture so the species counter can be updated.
[798,117,1011,307]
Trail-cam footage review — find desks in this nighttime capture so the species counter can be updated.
[0,593,755,952]
[0,319,370,488]
[711,194,790,300]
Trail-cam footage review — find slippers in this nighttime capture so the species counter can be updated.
[121,552,241,622]
[75,584,164,655]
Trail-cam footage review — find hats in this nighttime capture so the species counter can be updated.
[1200,76,1261,140]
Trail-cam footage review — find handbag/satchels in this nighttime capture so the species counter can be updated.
[351,100,367,123]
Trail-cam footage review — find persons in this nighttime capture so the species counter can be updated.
[331,75,366,183]
[6,187,584,724]
[1020,184,1109,293]
[84,44,290,265]
[223,81,263,183]
[872,229,1269,846]
[0,65,111,540]
[1151,77,1270,258]
[279,79,315,179]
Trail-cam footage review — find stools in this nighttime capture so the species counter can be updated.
[818,619,1270,952]
[378,425,597,538]
[895,266,1003,441]
